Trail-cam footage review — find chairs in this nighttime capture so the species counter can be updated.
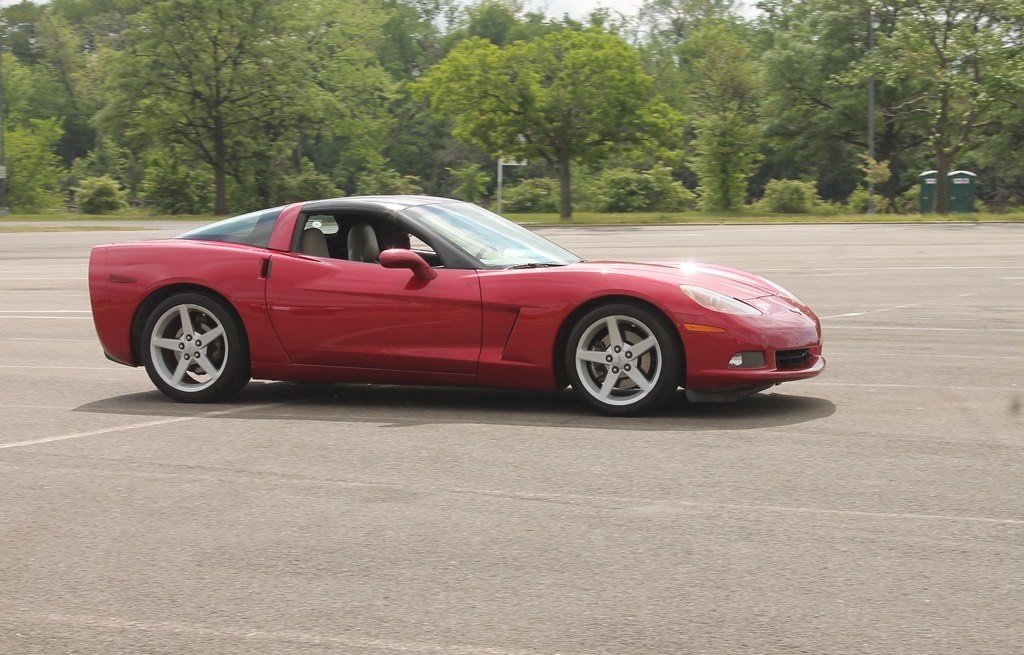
[346,221,380,264]
[301,228,330,258]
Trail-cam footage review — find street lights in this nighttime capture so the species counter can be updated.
[866,6,884,214]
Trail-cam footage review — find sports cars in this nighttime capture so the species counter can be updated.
[88,191,829,414]
